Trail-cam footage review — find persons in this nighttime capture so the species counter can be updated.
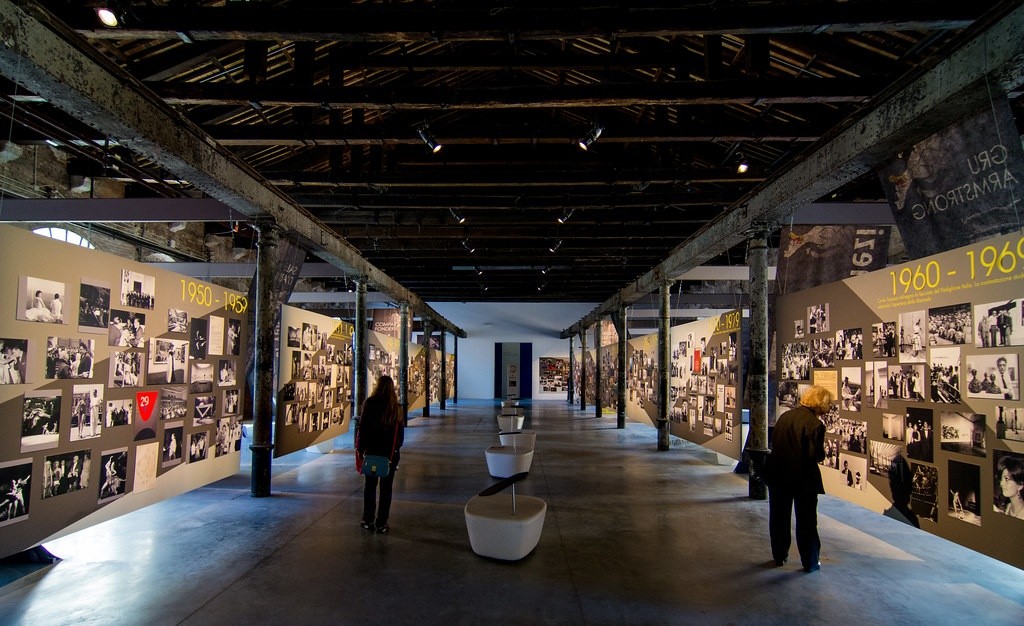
[542,295,1024,529]
[354,372,406,536]
[762,384,833,575]
[0,264,458,526]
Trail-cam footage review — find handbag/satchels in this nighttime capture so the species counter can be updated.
[358,455,391,476]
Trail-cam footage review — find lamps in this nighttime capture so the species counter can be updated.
[411,112,461,154]
[537,206,574,292]
[450,196,490,291]
[557,106,608,151]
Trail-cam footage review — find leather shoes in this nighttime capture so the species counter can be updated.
[802,561,822,573]
[774,558,789,565]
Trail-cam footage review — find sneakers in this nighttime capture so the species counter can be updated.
[375,522,390,535]
[359,519,376,532]
